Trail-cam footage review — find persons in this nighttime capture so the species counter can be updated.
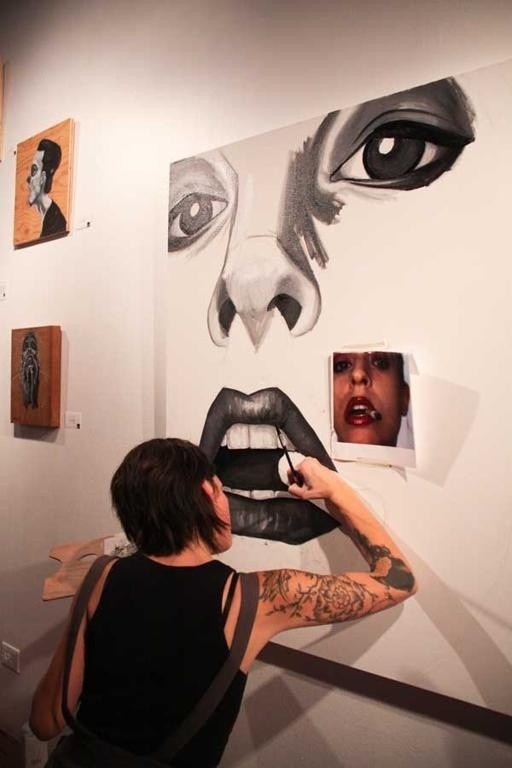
[335,351,411,446]
[17,331,42,409]
[167,78,475,550]
[29,437,418,767]
[26,138,67,238]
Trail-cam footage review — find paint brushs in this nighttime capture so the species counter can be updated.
[273,421,298,483]
